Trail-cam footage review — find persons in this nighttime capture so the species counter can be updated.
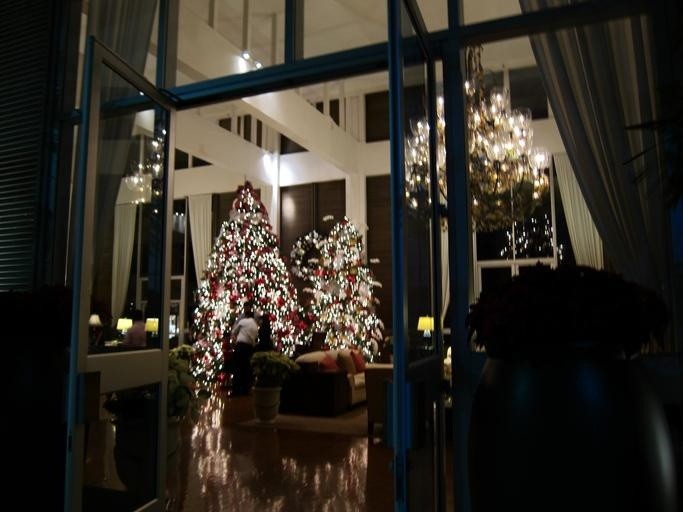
[230,302,262,396]
[122,309,147,346]
[95,311,119,347]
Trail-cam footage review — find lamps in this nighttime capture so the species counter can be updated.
[497,219,567,275]
[124,129,166,199]
[88,315,162,344]
[417,316,437,341]
[391,44,550,231]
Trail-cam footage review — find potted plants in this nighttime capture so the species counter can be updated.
[465,259,680,511]
[102,344,211,495]
[248,351,301,423]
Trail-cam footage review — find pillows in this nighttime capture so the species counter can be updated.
[350,350,368,373]
[337,349,357,374]
[319,350,343,374]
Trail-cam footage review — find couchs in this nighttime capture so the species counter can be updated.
[276,344,383,416]
